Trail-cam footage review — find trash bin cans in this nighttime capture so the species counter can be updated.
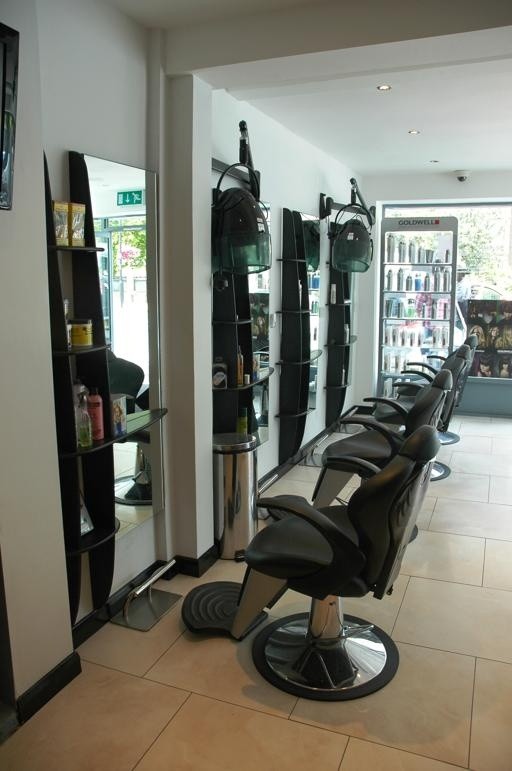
[213,434,260,562]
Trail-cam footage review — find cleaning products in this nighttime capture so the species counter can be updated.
[237,346,244,386]
[76,390,93,447]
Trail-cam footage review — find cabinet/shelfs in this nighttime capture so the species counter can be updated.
[377,216,458,397]
[323,223,358,429]
[215,272,253,437]
[41,151,115,627]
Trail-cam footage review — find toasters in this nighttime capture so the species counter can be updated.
[76,388,105,447]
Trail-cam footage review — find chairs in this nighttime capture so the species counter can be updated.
[310,326,482,545]
[182,424,441,701]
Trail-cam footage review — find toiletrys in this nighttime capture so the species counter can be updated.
[330,283,337,304]
[312,300,319,313]
[344,323,350,344]
[85,387,105,441]
[383,234,451,374]
[68,318,93,347]
[308,272,320,289]
[253,353,261,379]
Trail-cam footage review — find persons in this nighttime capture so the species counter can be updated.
[476,354,497,377]
[499,359,510,379]
[498,302,511,325]
[468,324,485,352]
[487,326,499,343]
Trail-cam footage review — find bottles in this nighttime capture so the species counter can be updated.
[311,299,320,313]
[235,407,249,443]
[211,356,228,390]
[236,344,244,387]
[307,264,320,289]
[344,323,350,343]
[67,317,93,350]
[330,283,337,306]
[380,230,453,401]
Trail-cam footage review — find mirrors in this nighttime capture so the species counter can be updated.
[249,201,321,451]
[80,153,166,542]
